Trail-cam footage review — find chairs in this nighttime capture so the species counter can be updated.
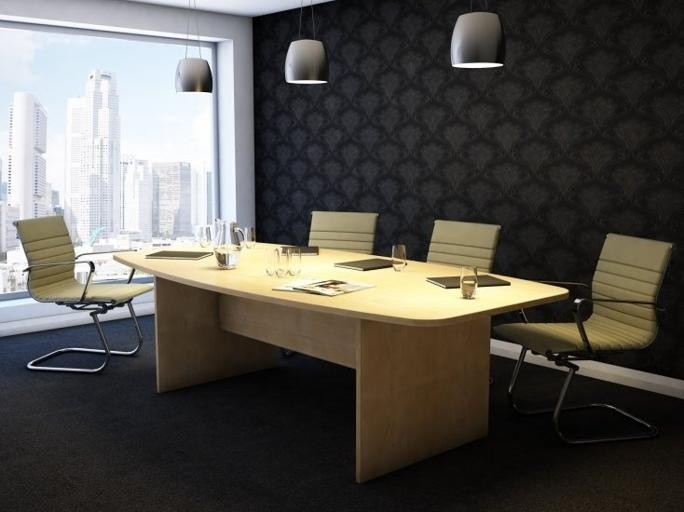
[308,210,380,254]
[13,215,155,372]
[427,219,503,273]
[491,232,673,445]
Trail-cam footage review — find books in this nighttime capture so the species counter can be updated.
[426,274,512,289]
[273,244,319,255]
[333,257,406,269]
[270,275,373,297]
[144,249,215,262]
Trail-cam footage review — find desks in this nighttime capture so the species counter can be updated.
[113,241,572,483]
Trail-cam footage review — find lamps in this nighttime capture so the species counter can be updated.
[451,1,504,69]
[284,0,329,84]
[174,0,213,93]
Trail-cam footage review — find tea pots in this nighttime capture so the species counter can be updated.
[214,217,246,270]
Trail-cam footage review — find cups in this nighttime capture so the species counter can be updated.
[459,264,479,298]
[245,226,256,248]
[199,226,221,251]
[391,245,408,273]
[262,247,302,279]
